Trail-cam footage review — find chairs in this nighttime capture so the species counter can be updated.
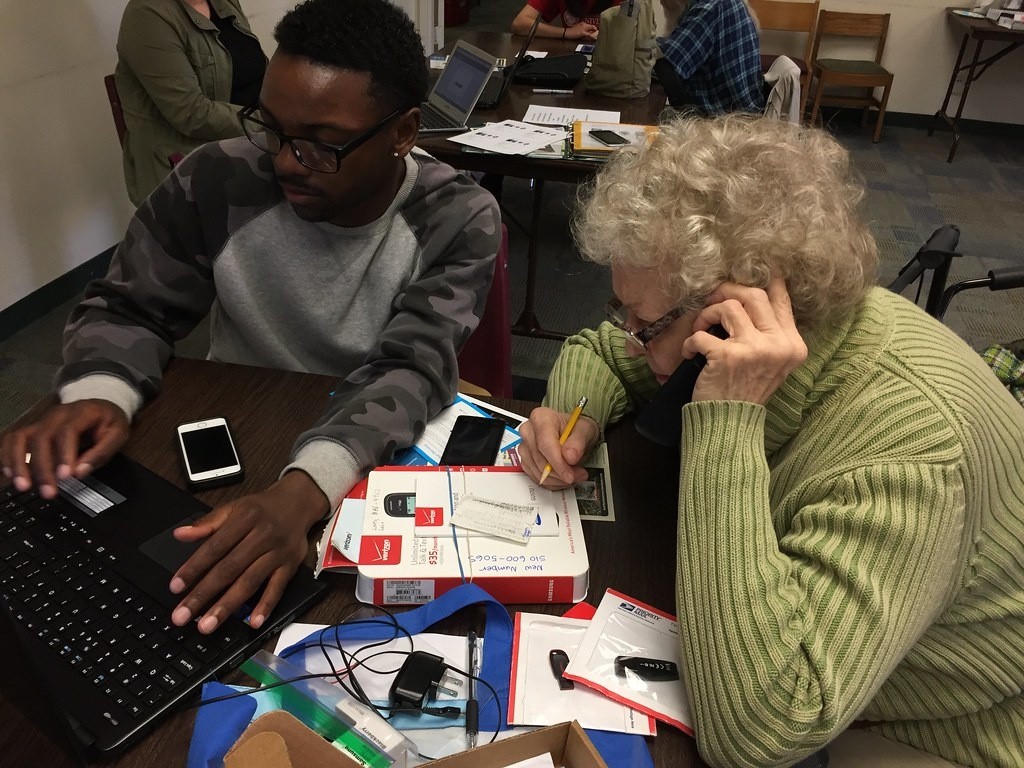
[457,223,513,399]
[748,0,894,143]
[937,266,1024,411]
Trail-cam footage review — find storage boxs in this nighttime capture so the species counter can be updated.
[313,466,590,605]
[223,710,610,768]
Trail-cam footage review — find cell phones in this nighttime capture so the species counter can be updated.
[580,44,595,54]
[589,130,631,147]
[176,417,245,492]
[631,322,731,448]
[385,493,416,517]
[438,414,505,466]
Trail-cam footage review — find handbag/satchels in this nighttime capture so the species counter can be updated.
[502,53,590,88]
[586,0,657,99]
[185,585,653,768]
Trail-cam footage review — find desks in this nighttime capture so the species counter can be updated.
[0,358,831,768]
[413,32,669,341]
[927,6,1024,162]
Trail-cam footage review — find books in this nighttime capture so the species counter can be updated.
[569,121,659,158]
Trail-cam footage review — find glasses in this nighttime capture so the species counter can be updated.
[237,94,408,174]
[604,293,702,352]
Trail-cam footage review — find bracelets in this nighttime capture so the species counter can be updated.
[561,28,566,38]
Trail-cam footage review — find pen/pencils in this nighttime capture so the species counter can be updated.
[466,626,478,749]
[541,396,589,487]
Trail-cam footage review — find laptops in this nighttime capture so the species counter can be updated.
[422,11,545,108]
[418,40,498,133]
[0,437,328,768]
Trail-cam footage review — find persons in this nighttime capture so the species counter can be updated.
[656,0,767,120]
[518,112,1024,768]
[0,0,503,635]
[114,0,270,208]
[511,0,626,40]
